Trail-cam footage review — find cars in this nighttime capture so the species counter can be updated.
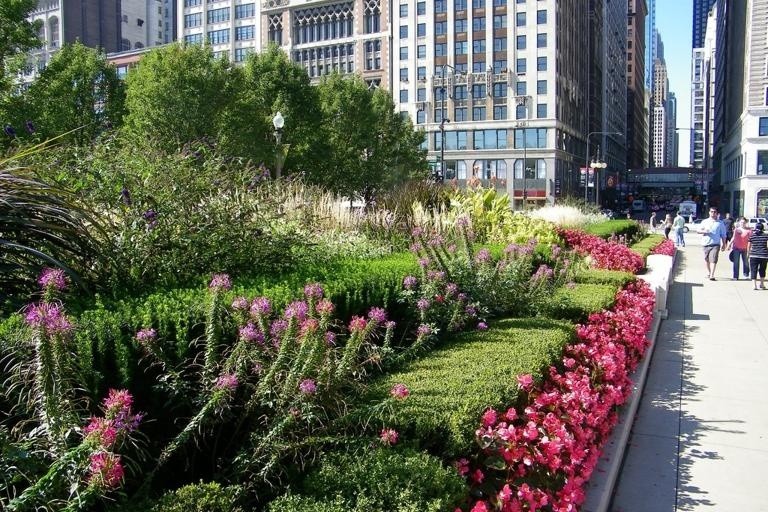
[734,218,768,231]
[650,198,679,212]
[602,207,630,218]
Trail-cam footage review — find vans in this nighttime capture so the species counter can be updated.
[632,200,647,211]
[679,199,698,221]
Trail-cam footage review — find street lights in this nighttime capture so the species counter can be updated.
[440,64,466,181]
[584,131,624,206]
[272,111,285,179]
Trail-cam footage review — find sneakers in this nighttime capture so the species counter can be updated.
[733,273,765,290]
[707,275,715,280]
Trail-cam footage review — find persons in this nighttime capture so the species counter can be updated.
[689,207,768,291]
[660,213,672,239]
[673,211,685,247]
[649,212,657,234]
